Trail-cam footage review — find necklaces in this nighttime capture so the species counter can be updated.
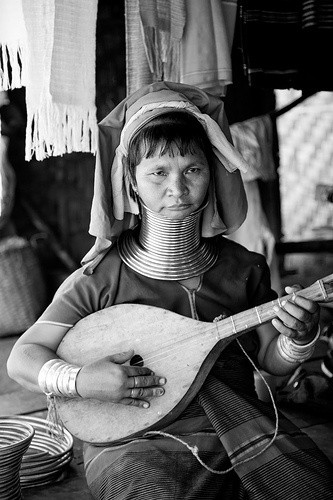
[112,200,223,283]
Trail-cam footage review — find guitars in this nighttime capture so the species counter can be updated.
[53,272,333,447]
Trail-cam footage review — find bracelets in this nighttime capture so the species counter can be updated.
[36,351,84,405]
[274,324,322,366]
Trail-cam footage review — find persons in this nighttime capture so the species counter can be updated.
[2,109,333,500]
[312,182,333,205]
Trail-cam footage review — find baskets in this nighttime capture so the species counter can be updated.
[1,236,46,338]
